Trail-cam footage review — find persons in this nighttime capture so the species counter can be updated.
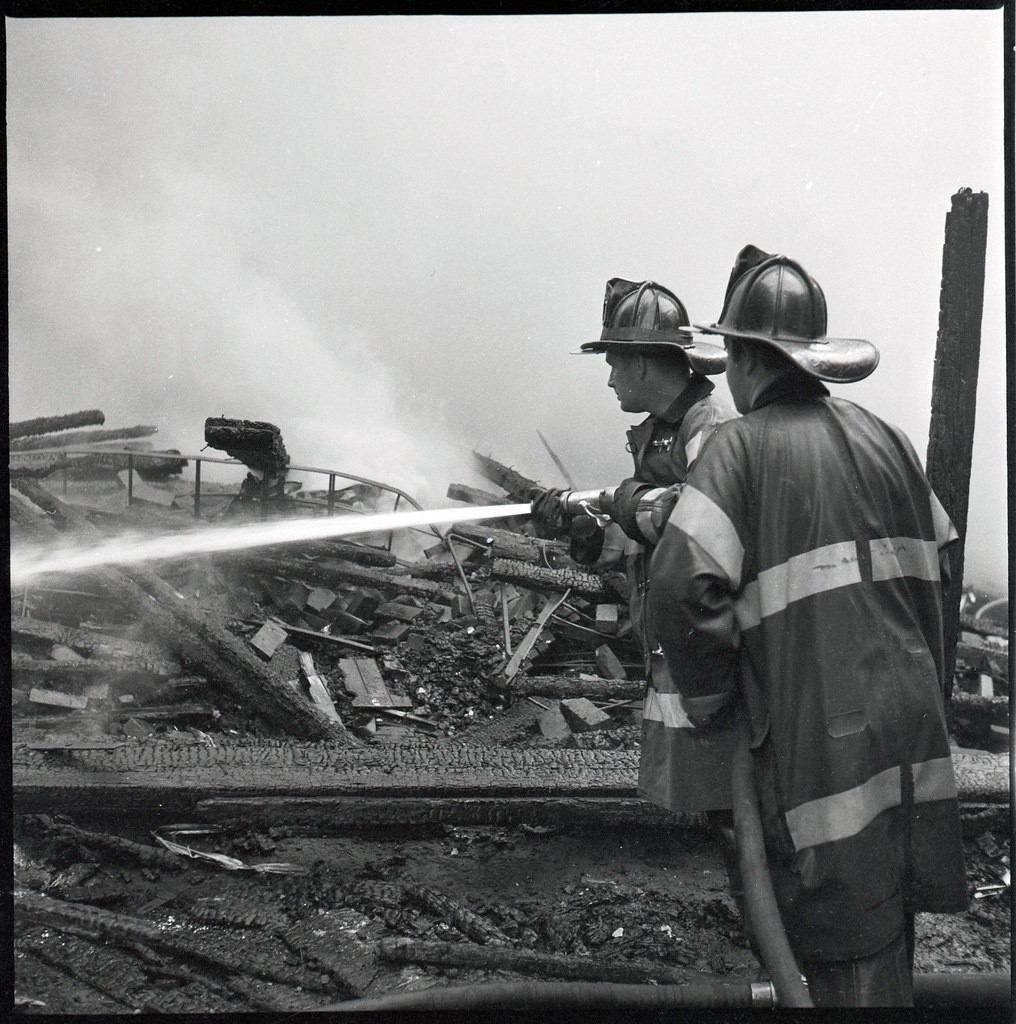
[527,278,739,812]
[647,244,971,1008]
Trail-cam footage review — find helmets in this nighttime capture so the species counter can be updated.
[693,246,879,384]
[580,279,725,378]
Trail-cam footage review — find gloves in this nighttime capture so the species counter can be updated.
[532,491,573,538]
